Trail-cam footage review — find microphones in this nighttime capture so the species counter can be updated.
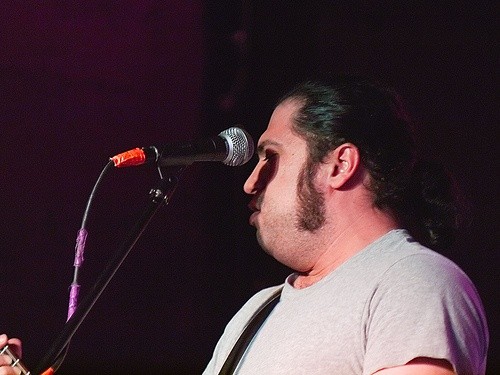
[111,126,254,169]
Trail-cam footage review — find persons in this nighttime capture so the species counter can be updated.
[1,70,490,375]
